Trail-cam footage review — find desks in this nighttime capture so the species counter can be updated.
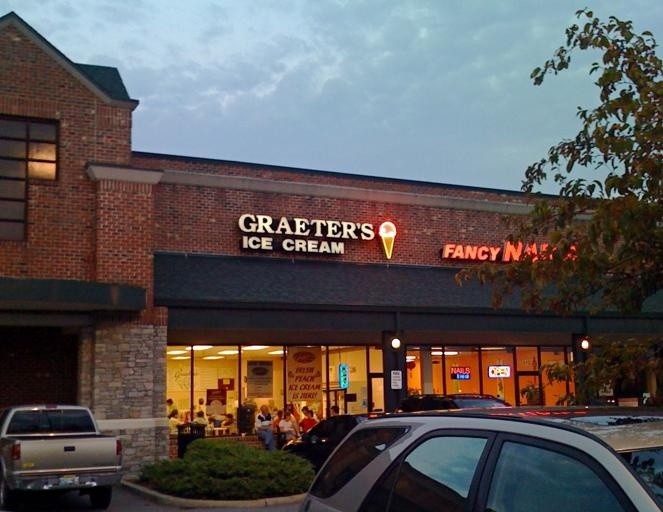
[206,427,227,436]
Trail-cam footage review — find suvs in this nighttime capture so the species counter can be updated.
[395,391,511,415]
[295,409,663,512]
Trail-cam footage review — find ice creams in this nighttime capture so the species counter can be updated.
[379,220,397,260]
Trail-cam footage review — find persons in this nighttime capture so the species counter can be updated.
[166,398,338,450]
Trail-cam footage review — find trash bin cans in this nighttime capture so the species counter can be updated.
[176,424,207,458]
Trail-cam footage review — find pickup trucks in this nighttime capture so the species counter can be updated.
[0,405,124,511]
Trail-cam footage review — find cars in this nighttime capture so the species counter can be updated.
[279,412,387,473]
